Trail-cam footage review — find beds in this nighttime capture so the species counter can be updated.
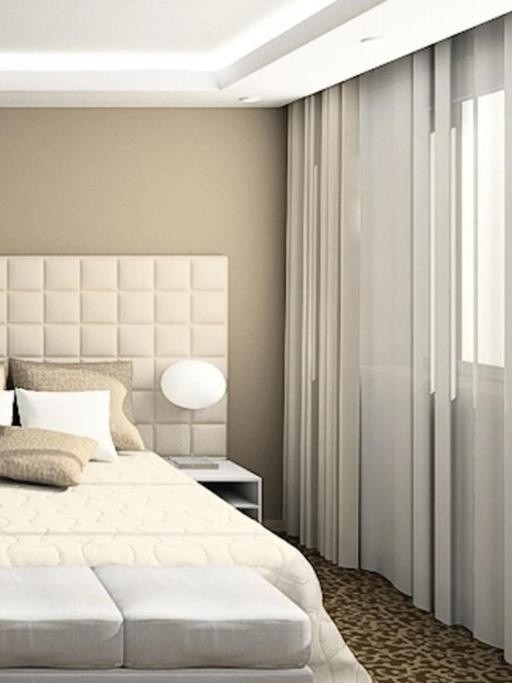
[0,452,375,683]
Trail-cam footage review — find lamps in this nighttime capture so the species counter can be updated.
[159,357,228,471]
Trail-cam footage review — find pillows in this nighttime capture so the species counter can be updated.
[9,357,147,454]
[0,360,8,391]
[13,386,123,466]
[0,389,16,426]
[0,424,99,490]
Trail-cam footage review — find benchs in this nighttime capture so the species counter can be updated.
[0,562,316,683]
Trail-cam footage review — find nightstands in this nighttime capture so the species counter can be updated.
[181,459,264,526]
